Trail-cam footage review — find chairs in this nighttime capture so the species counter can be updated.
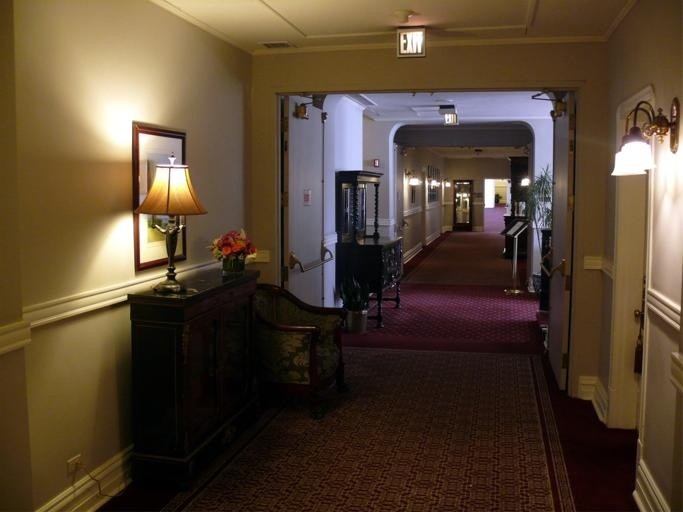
[246,285,350,421]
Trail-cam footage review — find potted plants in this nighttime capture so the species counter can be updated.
[521,162,554,299]
[339,275,371,336]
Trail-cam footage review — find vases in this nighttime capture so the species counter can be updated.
[219,250,246,281]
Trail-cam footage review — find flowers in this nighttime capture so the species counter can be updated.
[207,228,256,273]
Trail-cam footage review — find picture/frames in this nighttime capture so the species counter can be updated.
[130,119,190,274]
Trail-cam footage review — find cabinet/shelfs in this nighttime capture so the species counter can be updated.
[127,269,263,492]
[334,169,403,330]
[451,179,474,231]
[502,153,528,260]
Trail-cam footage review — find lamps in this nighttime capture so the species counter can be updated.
[392,27,427,59]
[610,96,680,176]
[134,153,208,294]
[437,107,459,128]
